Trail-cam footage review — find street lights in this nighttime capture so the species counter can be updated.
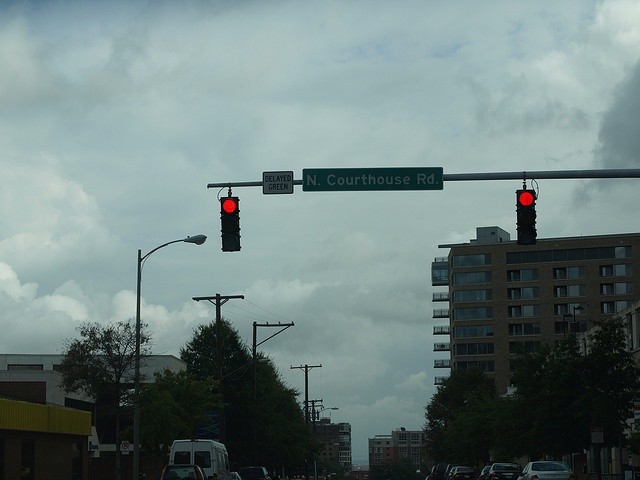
[315,407,338,414]
[131,234,208,480]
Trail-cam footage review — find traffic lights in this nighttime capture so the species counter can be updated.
[217,186,243,253]
[515,180,538,245]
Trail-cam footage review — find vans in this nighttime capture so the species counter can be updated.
[169,438,228,480]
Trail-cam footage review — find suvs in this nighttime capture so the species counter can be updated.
[161,464,206,480]
[239,466,267,480]
[454,467,475,480]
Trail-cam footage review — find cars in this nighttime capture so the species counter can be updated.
[518,460,575,480]
[489,463,521,480]
